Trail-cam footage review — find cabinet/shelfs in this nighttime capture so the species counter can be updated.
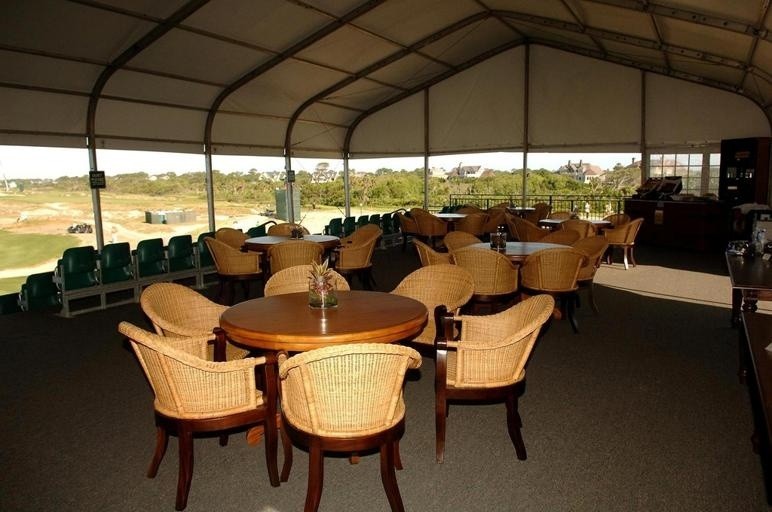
[718,136,772,205]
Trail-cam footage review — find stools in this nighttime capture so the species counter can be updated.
[724,249,772,385]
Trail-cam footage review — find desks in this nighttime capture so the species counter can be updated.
[738,312,772,454]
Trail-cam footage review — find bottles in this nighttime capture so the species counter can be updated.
[744,168,752,179]
[727,168,735,178]
[753,227,764,255]
